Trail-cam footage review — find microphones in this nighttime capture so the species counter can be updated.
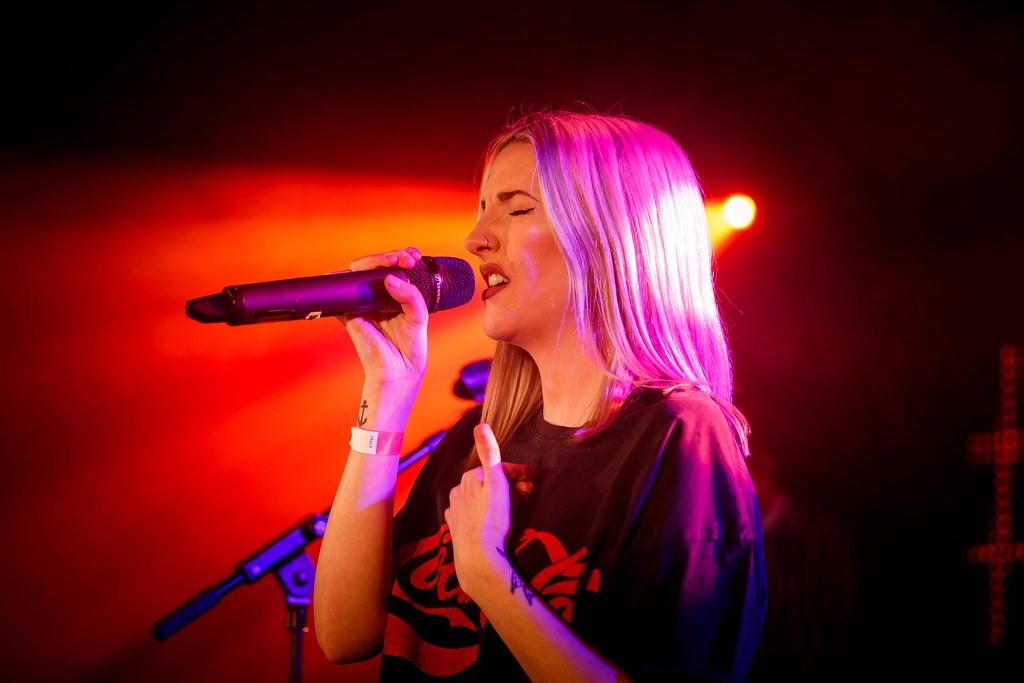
[186,255,476,327]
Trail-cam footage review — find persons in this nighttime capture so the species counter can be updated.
[314,111,769,683]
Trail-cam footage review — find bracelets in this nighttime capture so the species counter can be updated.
[349,426,405,456]
